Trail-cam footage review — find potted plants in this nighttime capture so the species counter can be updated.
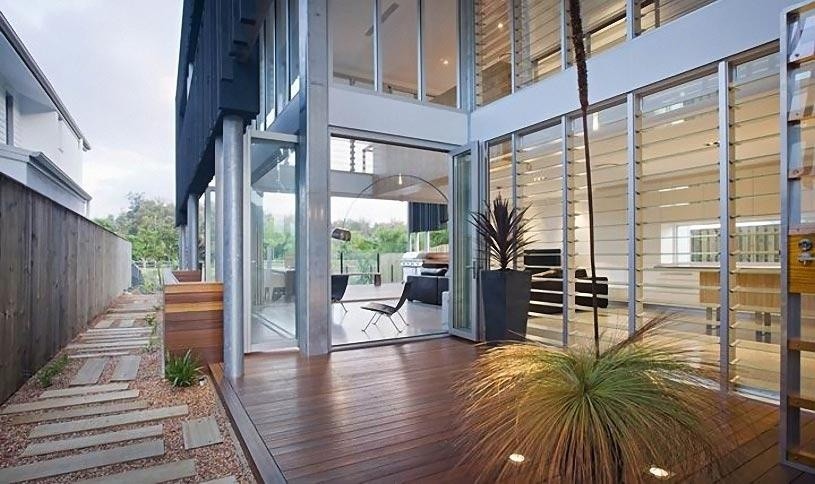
[462,192,543,343]
[441,307,764,482]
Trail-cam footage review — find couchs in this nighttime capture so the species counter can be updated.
[407,265,448,305]
[523,247,608,313]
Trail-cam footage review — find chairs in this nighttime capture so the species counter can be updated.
[330,274,349,313]
[359,280,415,333]
